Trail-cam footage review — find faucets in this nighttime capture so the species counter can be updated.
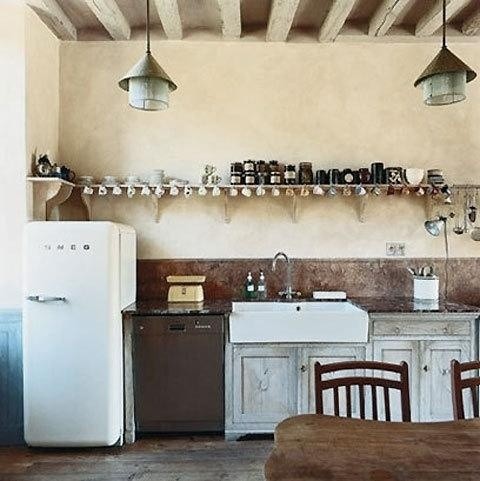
[271,252,302,300]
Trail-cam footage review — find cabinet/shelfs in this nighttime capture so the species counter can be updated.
[225,344,366,442]
[25,175,478,220]
[370,313,480,422]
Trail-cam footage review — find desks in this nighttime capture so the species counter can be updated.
[265,415,480,480]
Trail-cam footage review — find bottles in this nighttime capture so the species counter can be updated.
[245,273,256,299]
[270,172,280,184]
[269,161,279,172]
[244,161,254,173]
[258,173,268,184]
[284,165,295,184]
[245,173,255,184]
[256,161,266,173]
[231,173,241,184]
[299,162,312,184]
[231,162,242,172]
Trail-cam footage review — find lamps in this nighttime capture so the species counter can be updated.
[120,2,177,109]
[413,0,476,107]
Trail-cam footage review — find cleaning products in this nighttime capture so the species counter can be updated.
[243,269,258,299]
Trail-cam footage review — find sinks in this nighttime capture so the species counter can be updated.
[228,302,370,344]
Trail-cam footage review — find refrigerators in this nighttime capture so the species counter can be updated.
[19,219,137,451]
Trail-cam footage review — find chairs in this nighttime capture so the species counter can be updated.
[452,359,478,418]
[312,361,410,422]
